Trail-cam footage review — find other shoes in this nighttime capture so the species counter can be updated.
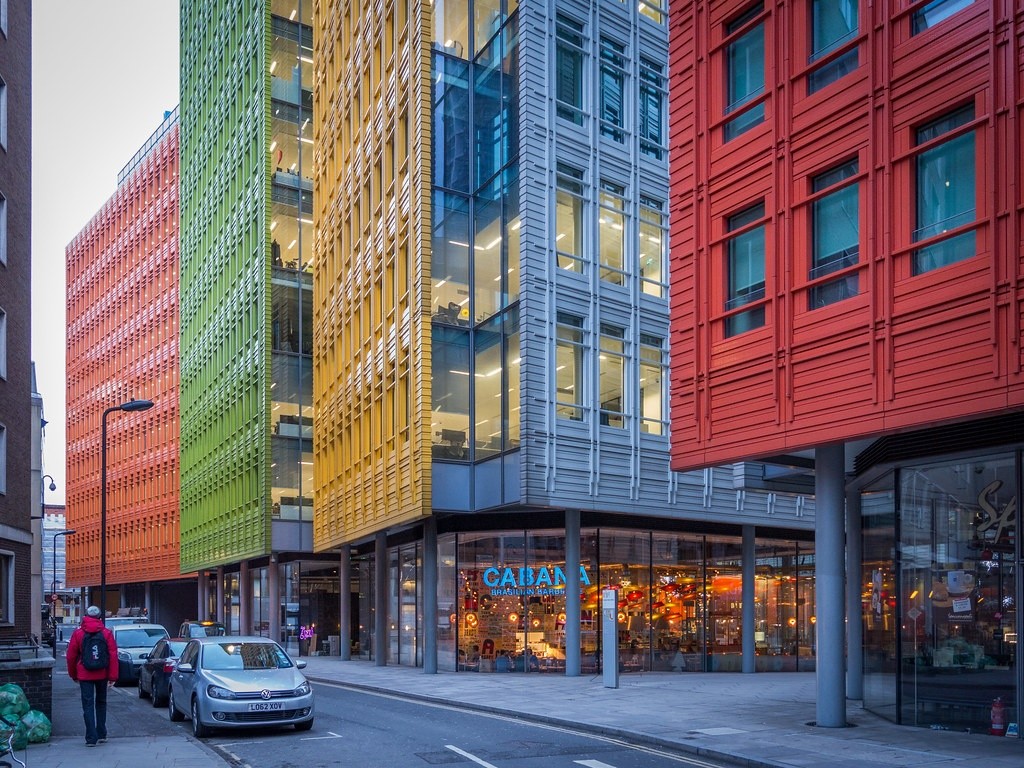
[86,742,96,747]
[98,738,109,743]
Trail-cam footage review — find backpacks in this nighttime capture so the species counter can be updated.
[76,632,110,670]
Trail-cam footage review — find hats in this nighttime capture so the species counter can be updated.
[85,606,108,619]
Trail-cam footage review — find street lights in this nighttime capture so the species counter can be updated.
[51,580,62,595]
[54,530,76,658]
[101,397,155,625]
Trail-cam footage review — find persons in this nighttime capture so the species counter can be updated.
[629,639,771,671]
[67,606,118,747]
[459,644,539,673]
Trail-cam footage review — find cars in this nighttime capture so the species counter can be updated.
[138,638,196,707]
[169,636,315,736]
[105,616,170,686]
[178,621,228,638]
